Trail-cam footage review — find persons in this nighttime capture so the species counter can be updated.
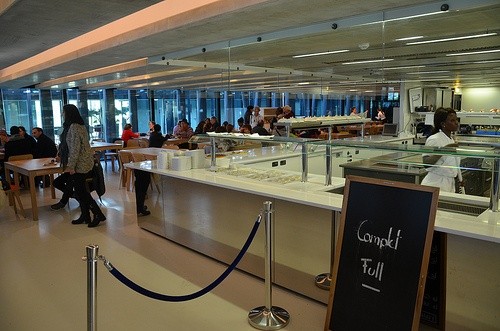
[173,105,338,149]
[420,107,467,194]
[51,104,106,228]
[148,124,171,148]
[30,127,58,188]
[0,126,36,190]
[134,169,151,215]
[376,108,386,124]
[350,106,362,116]
[121,124,139,147]
[147,122,156,136]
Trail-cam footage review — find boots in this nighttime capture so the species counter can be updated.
[71,199,91,224]
[88,200,106,227]
[136,181,150,215]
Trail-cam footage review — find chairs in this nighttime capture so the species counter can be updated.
[8,139,179,194]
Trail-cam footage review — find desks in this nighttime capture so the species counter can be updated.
[4,158,64,221]
[121,147,180,155]
[90,141,122,172]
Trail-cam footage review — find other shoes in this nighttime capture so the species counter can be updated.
[50,202,65,211]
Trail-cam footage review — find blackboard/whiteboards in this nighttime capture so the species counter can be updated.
[323,173,440,331]
